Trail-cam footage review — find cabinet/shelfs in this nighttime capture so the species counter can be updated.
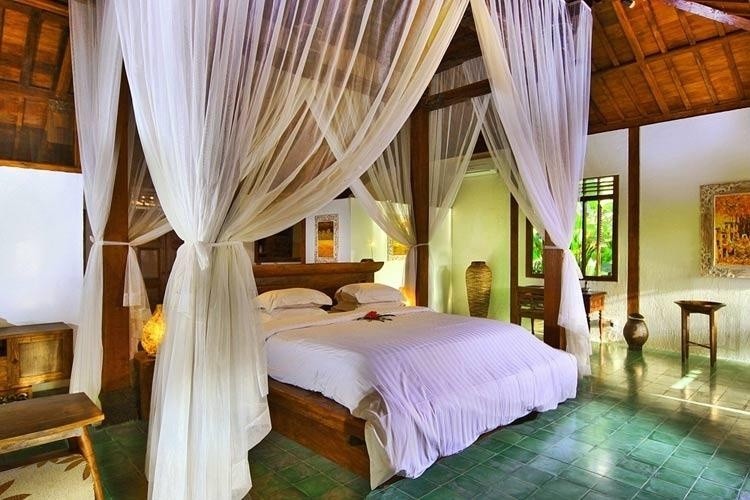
[133,231,183,313]
[0,322,73,401]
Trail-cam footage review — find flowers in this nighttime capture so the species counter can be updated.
[358,311,396,322]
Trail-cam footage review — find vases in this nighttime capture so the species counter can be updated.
[623,313,649,351]
[466,261,492,317]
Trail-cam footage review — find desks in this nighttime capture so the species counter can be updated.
[517,286,607,343]
[0,393,107,500]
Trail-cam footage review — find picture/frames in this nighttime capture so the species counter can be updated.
[387,214,411,261]
[700,180,750,279]
[314,214,339,263]
[255,227,293,264]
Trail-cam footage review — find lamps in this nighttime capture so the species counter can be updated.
[141,304,165,357]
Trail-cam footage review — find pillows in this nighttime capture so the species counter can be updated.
[256,283,404,319]
[253,262,578,491]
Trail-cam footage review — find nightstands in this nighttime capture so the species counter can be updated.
[134,351,155,422]
